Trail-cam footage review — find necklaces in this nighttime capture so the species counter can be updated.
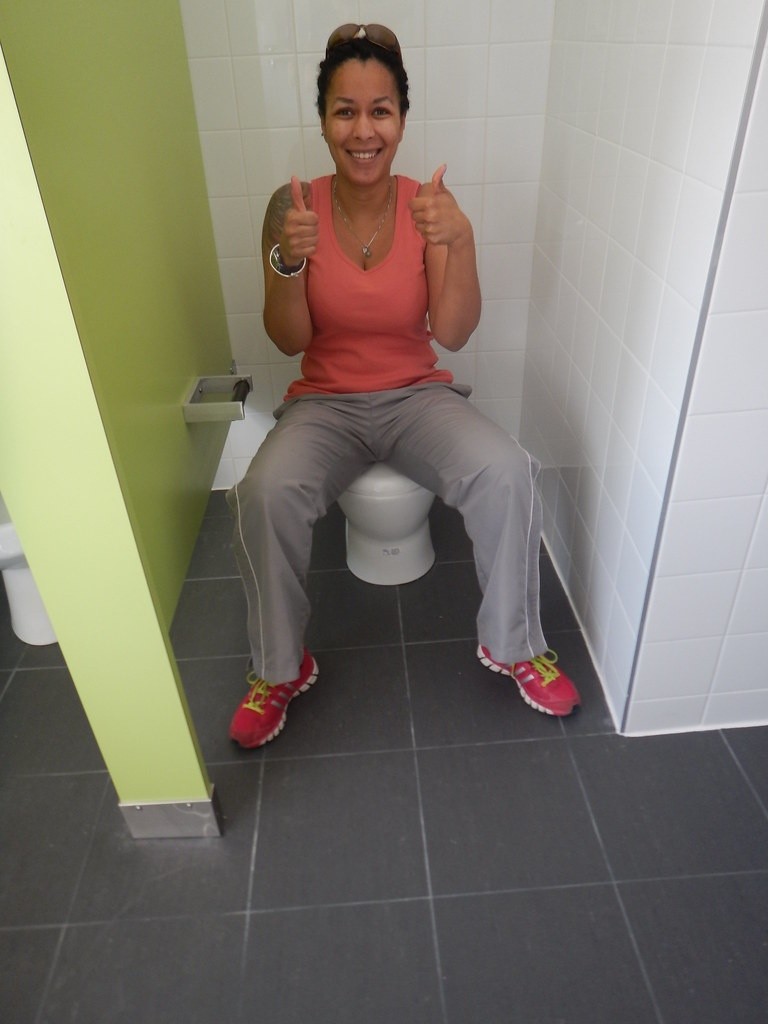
[333,177,393,258]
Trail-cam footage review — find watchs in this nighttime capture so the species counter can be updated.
[272,247,305,276]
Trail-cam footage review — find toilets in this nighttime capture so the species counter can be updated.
[335,461,438,587]
[0,522,57,647]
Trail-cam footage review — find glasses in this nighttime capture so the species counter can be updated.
[326,23,401,59]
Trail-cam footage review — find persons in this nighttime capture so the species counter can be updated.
[227,24,582,750]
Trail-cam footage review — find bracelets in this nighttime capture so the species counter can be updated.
[270,244,306,278]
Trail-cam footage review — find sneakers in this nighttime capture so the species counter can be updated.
[229,649,318,748]
[475,644,581,716]
